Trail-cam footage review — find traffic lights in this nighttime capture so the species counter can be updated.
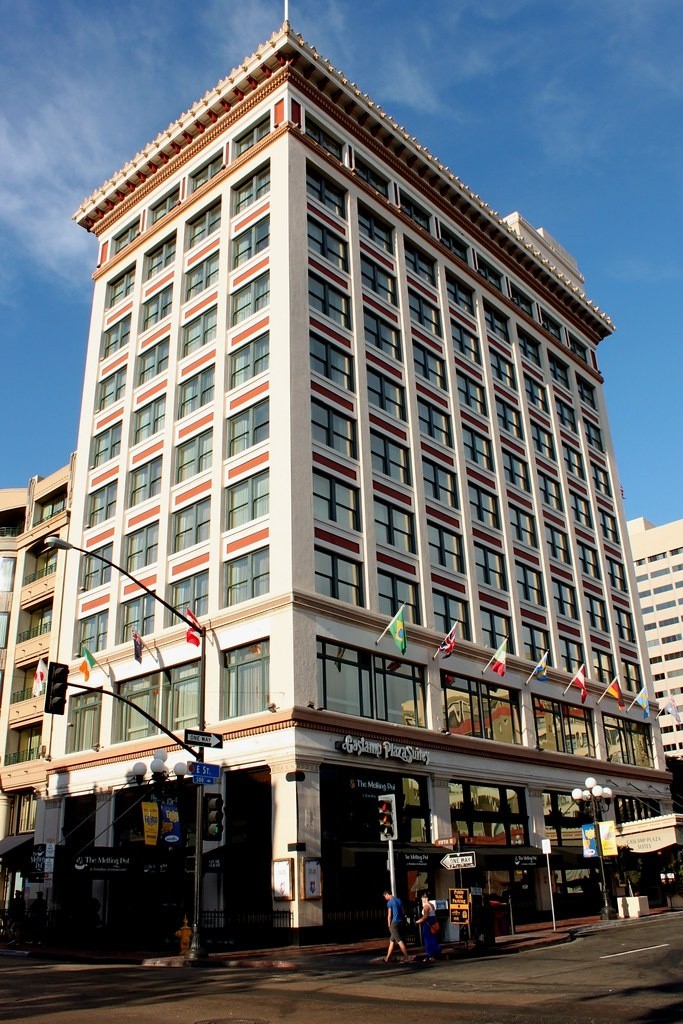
[45,662,69,715]
[204,793,223,842]
[377,794,399,842]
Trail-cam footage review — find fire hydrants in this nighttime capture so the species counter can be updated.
[174,913,193,955]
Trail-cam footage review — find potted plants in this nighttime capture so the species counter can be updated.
[613,849,649,919]
[662,859,683,909]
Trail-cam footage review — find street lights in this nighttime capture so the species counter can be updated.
[42,535,207,964]
[572,776,618,920]
[132,749,188,954]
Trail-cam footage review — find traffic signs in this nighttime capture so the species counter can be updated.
[441,851,477,870]
[184,729,224,750]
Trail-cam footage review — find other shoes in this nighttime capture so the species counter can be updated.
[404,959,412,964]
[383,958,388,963]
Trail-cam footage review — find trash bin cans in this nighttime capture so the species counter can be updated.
[488,901,510,937]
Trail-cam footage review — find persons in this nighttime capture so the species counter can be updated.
[8,891,47,945]
[413,897,442,961]
[383,891,411,962]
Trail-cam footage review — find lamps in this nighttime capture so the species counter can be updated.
[44,755,52,760]
[93,743,100,752]
[269,704,276,713]
[607,754,613,762]
[536,746,544,752]
[442,730,451,735]
[306,701,314,707]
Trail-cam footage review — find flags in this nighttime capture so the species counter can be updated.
[247,645,260,656]
[491,640,506,676]
[78,648,95,681]
[438,623,456,659]
[535,654,548,682]
[665,697,681,723]
[186,608,200,647]
[132,631,143,663]
[637,688,649,719]
[32,660,48,696]
[573,666,587,703]
[388,610,407,655]
[608,678,625,711]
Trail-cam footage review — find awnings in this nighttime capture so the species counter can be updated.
[32,847,131,880]
[0,834,33,856]
[342,843,454,868]
[554,845,619,861]
[459,844,560,868]
